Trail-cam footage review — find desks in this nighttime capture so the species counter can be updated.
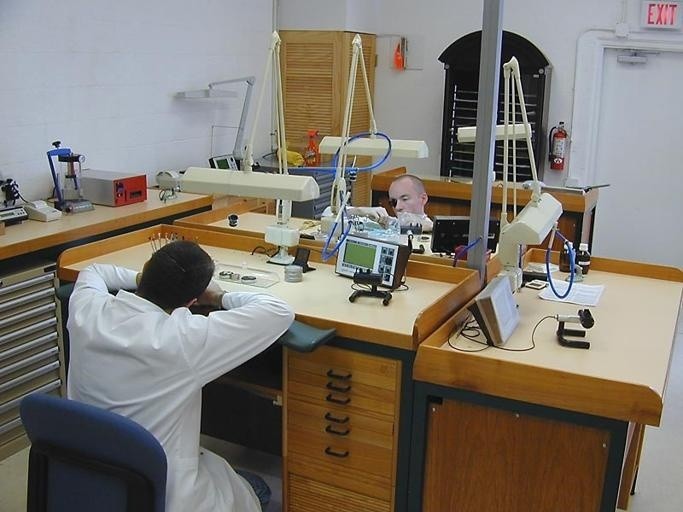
[370,166,598,255]
[411,248,683,512]
[57,224,481,512]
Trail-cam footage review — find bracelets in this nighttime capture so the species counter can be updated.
[218,290,229,310]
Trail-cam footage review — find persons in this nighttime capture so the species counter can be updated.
[65,238,297,512]
[320,174,433,233]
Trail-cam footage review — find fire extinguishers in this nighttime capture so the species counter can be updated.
[548,122,567,170]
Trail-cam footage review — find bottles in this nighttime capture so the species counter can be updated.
[575,243,591,274]
[559,241,573,272]
